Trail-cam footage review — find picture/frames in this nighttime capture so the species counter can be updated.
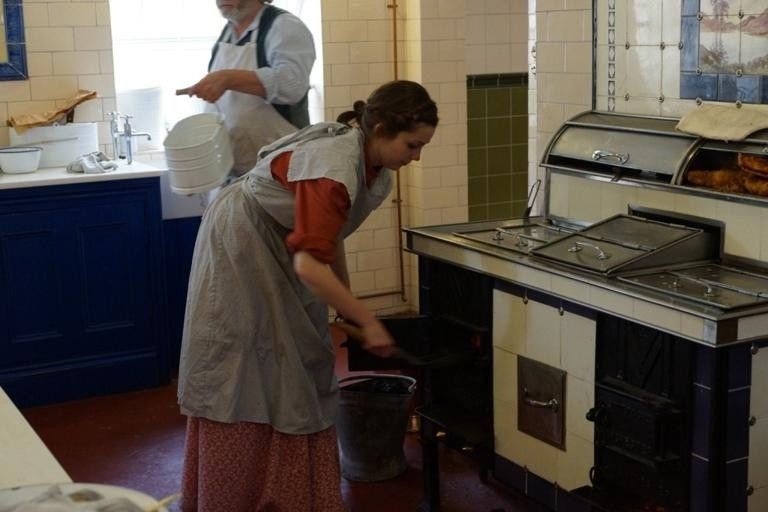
[0,0,31,81]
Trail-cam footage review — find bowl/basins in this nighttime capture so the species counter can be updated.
[0,146,42,175]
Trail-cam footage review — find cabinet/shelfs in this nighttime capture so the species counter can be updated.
[0,176,203,410]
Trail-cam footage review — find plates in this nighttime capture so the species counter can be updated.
[1,483,167,512]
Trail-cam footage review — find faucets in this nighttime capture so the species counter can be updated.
[105,110,137,160]
[121,114,151,165]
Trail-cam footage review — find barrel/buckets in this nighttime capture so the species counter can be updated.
[334,373,419,484]
[163,86,235,196]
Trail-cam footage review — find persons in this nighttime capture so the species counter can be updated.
[186,1,316,213]
[176,79,438,512]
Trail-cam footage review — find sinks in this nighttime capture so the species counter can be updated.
[132,150,212,220]
